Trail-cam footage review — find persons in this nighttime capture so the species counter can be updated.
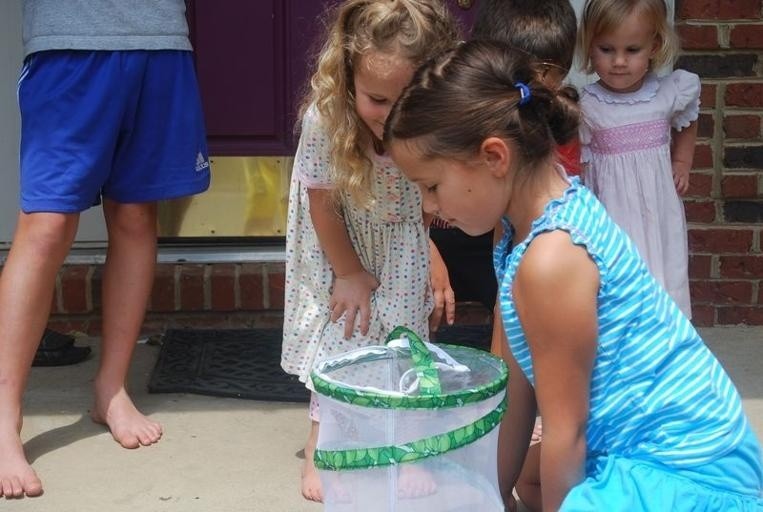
[581,0,701,322]
[1,2,211,497]
[383,39,757,512]
[427,0,581,353]
[282,0,452,501]
